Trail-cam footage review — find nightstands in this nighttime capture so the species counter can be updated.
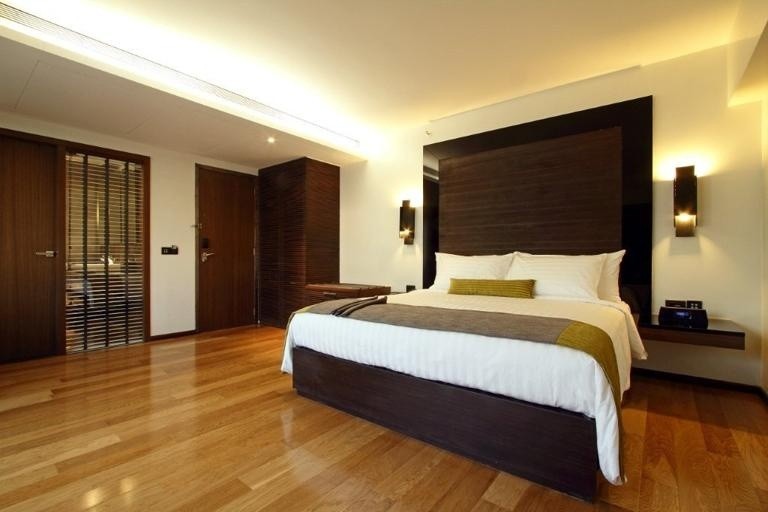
[306,283,391,305]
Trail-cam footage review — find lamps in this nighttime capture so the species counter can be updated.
[673,165,698,238]
[399,199,415,244]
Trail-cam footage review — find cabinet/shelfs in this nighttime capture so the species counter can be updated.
[259,155,340,329]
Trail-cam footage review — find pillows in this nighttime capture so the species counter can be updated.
[507,251,607,301]
[597,250,626,302]
[426,252,514,289]
[446,277,538,300]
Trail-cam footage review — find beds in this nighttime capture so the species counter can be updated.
[281,94,653,504]
